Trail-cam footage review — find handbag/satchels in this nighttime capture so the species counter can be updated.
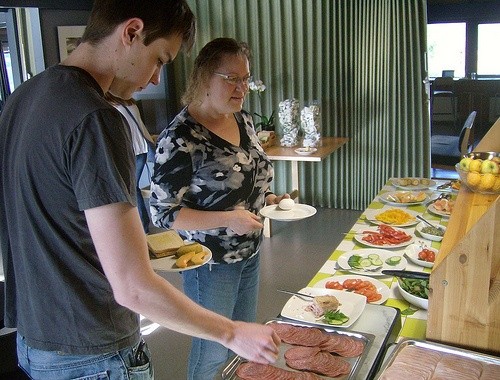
[144,137,156,163]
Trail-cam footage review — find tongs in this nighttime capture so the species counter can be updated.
[426,193,452,205]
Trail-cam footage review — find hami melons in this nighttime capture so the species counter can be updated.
[175,250,208,267]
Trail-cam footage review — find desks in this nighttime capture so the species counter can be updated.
[263,137,349,238]
[429,78,500,131]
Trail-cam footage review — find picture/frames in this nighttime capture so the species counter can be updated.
[57,26,87,62]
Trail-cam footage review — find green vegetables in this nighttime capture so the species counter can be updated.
[399,277,430,300]
[324,309,350,321]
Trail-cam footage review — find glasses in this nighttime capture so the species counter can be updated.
[214,72,254,85]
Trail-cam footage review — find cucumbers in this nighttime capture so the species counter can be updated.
[348,254,401,268]
[328,318,348,324]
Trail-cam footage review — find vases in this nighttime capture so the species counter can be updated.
[257,131,277,150]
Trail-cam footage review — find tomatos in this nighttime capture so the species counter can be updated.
[363,224,412,246]
[418,248,435,262]
[324,279,382,302]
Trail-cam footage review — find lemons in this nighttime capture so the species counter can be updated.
[468,172,500,193]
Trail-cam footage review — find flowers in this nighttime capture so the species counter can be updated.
[247,80,275,132]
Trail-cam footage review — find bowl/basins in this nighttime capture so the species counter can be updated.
[455,163,500,195]
[416,221,447,241]
[405,244,439,267]
[397,271,431,309]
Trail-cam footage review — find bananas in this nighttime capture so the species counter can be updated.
[175,242,203,256]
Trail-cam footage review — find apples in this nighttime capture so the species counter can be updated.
[460,157,500,177]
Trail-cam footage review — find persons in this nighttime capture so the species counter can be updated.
[105,91,156,237]
[149,39,291,380]
[0,0,281,380]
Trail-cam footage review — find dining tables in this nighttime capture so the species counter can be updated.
[306,176,459,346]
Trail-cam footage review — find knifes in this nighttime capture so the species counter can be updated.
[277,288,341,306]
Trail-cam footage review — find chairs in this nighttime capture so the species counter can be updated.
[431,78,459,137]
[431,111,477,178]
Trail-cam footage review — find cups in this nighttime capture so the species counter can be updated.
[470,73,477,80]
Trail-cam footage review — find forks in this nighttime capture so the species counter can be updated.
[334,267,382,272]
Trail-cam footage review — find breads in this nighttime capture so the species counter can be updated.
[146,230,186,257]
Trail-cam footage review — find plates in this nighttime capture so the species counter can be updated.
[312,275,391,305]
[281,286,367,328]
[150,243,213,271]
[428,202,451,217]
[260,204,317,221]
[392,177,435,190]
[294,148,317,156]
[338,248,407,275]
[365,209,421,227]
[378,190,427,206]
[354,226,415,248]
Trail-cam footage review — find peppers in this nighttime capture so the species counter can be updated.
[375,209,416,224]
